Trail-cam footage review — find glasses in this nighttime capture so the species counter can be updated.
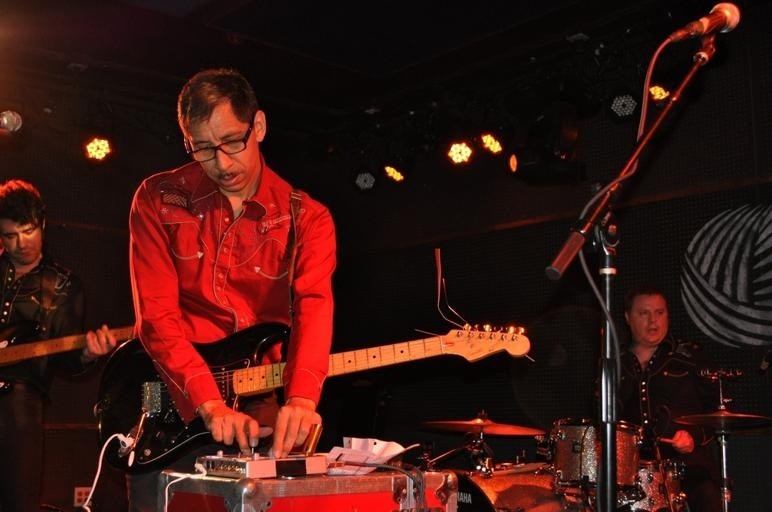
[184,125,252,162]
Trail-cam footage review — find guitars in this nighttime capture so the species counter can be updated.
[0,326,140,395]
[97,323,530,476]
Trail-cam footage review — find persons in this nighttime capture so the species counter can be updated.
[125,68,342,511]
[0,177,117,511]
[588,284,736,512]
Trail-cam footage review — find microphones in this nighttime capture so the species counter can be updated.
[671,3,741,42]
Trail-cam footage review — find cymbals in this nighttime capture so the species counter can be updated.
[671,411,769,428]
[424,419,542,438]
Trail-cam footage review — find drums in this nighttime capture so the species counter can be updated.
[456,464,597,512]
[553,418,644,495]
[617,458,687,510]
